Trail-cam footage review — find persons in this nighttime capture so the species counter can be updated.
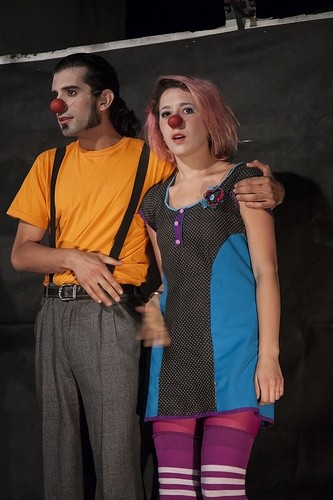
[4,52,287,500]
[133,72,288,500]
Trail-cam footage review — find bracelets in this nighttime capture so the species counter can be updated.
[148,291,163,299]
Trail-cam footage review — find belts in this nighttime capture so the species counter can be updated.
[42,285,136,300]
